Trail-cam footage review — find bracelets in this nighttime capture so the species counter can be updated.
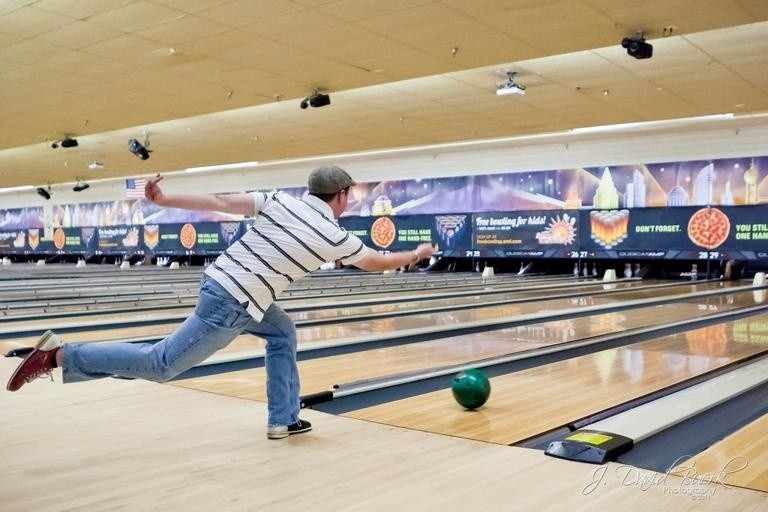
[409,249,420,263]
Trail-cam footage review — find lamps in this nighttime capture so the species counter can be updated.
[497,71,525,96]
[89,161,104,169]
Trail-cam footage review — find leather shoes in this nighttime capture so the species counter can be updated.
[266,419,311,439]
[6,329,64,393]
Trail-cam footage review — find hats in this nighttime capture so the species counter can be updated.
[308,164,358,195]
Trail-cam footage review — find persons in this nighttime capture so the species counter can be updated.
[6,164,438,439]
